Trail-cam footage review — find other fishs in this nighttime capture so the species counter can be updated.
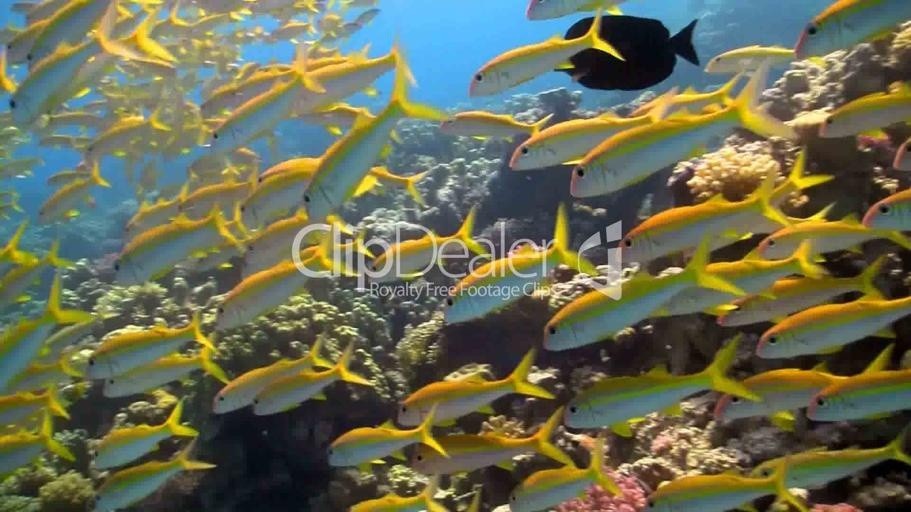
[0,0,910,510]
